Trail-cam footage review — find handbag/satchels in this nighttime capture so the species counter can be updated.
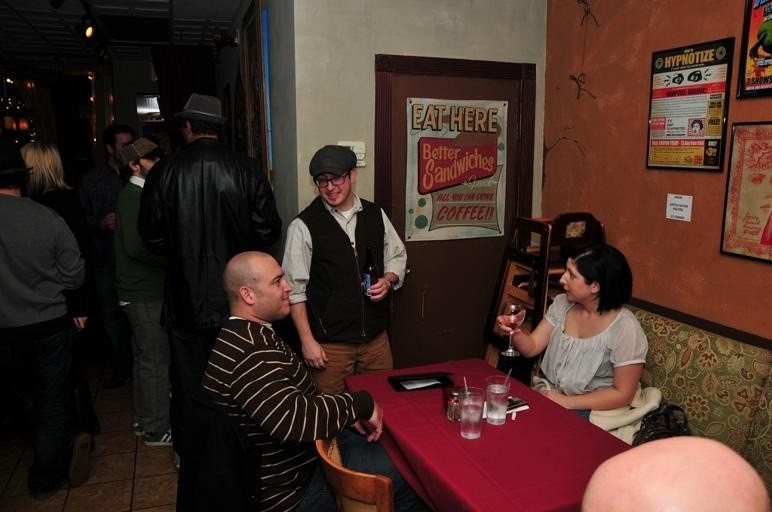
[631,403,691,447]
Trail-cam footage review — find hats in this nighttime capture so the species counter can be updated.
[310,145,357,180]
[172,93,228,125]
[118,136,159,166]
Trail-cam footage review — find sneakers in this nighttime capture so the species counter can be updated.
[133,421,146,434]
[144,431,173,446]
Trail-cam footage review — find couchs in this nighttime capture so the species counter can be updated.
[619,296,770,509]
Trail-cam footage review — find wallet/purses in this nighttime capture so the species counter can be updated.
[388,372,456,392]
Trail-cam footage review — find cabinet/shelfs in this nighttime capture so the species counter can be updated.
[485,217,606,387]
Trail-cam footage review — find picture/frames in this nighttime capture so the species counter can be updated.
[719,121,772,264]
[239,0,270,183]
[646,36,736,172]
[736,1,772,100]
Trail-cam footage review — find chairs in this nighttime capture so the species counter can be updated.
[165,329,269,512]
[313,436,396,511]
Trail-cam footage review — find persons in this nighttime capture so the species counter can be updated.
[140,94,281,414]
[280,146,408,394]
[0,139,88,497]
[203,251,426,512]
[17,142,100,449]
[112,136,174,446]
[74,125,135,390]
[498,244,648,445]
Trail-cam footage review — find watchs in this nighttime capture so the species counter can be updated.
[383,278,395,289]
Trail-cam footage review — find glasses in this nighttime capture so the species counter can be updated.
[314,169,351,188]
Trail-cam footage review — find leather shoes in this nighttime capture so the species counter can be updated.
[103,373,127,389]
[33,491,58,501]
[56,429,92,489]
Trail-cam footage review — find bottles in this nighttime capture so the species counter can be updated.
[362,265,377,297]
[446,391,461,423]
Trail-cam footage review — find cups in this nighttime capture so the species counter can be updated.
[459,386,485,440]
[484,374,510,426]
[499,301,526,358]
